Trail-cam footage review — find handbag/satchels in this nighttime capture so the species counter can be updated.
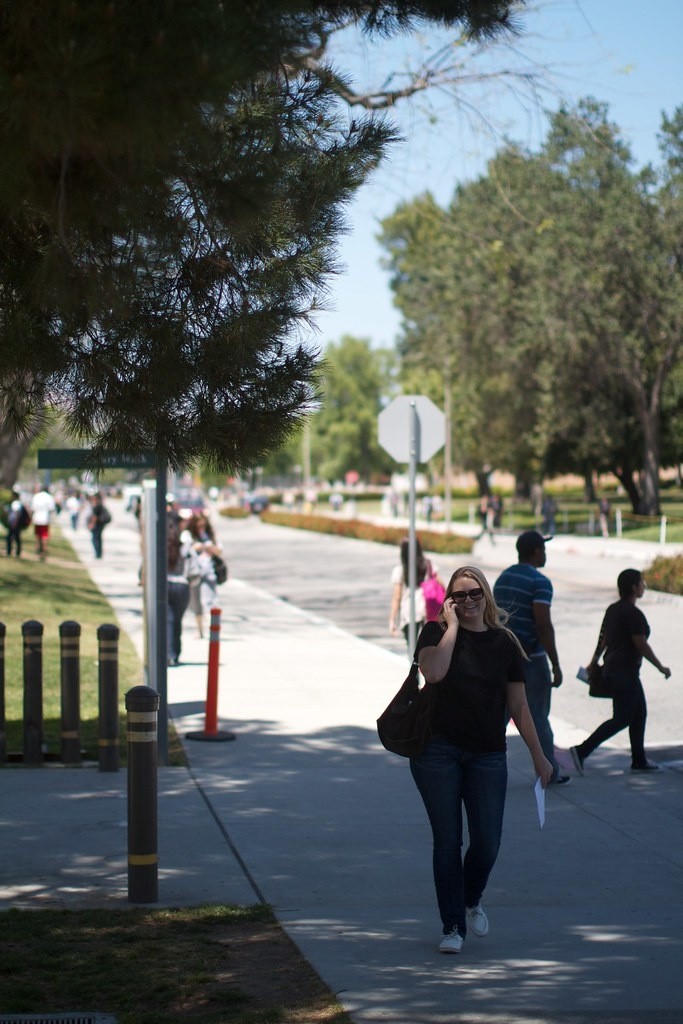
[377,620,448,757]
[421,579,445,620]
[589,665,613,698]
[211,555,226,584]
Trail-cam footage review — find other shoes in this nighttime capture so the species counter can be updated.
[547,775,571,786]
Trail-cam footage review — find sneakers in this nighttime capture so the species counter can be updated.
[570,747,584,775]
[630,761,661,772]
[466,906,488,937]
[439,925,464,953]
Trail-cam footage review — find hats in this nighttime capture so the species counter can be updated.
[516,531,553,551]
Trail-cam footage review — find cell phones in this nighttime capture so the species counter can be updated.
[449,602,461,619]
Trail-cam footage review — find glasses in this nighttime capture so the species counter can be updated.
[450,588,483,603]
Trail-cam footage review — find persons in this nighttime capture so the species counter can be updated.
[493,531,570,786]
[387,538,441,662]
[596,494,617,540]
[537,489,564,537]
[382,478,449,524]
[139,494,225,669]
[571,569,672,775]
[472,487,505,548]
[405,565,553,953]
[3,471,112,560]
